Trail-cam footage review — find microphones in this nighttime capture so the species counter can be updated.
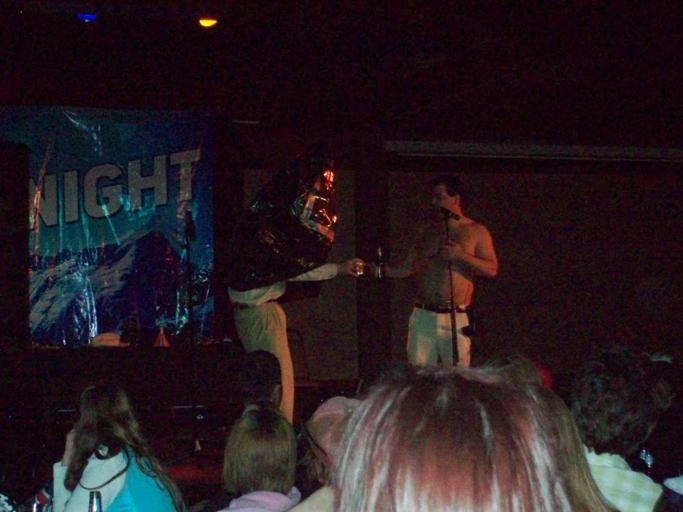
[436,205,460,220]
[186,212,196,239]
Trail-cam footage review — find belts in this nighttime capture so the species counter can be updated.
[234,300,276,309]
[413,302,466,313]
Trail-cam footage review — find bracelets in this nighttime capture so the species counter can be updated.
[458,252,470,262]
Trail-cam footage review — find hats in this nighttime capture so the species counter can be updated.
[310,395,361,452]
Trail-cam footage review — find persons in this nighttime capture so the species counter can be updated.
[366,175,499,368]
[17,348,681,511]
[226,134,365,427]
